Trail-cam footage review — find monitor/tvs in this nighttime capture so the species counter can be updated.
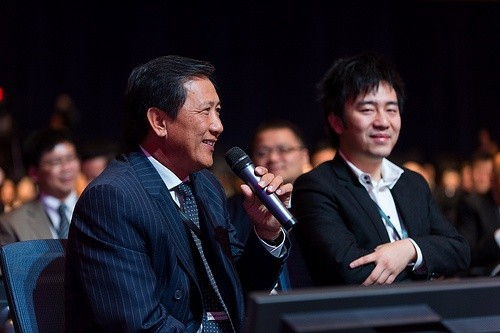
[246,276,500,333]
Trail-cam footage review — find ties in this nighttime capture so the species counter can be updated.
[58,204,70,239]
[171,181,235,333]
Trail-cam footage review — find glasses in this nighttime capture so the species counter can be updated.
[255,145,305,159]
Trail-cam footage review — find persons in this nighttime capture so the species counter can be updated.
[67,55,293,333]
[0,118,500,277]
[291,55,472,288]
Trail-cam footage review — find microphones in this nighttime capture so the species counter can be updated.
[225,147,298,232]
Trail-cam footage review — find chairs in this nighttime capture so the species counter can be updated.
[0,239,69,333]
